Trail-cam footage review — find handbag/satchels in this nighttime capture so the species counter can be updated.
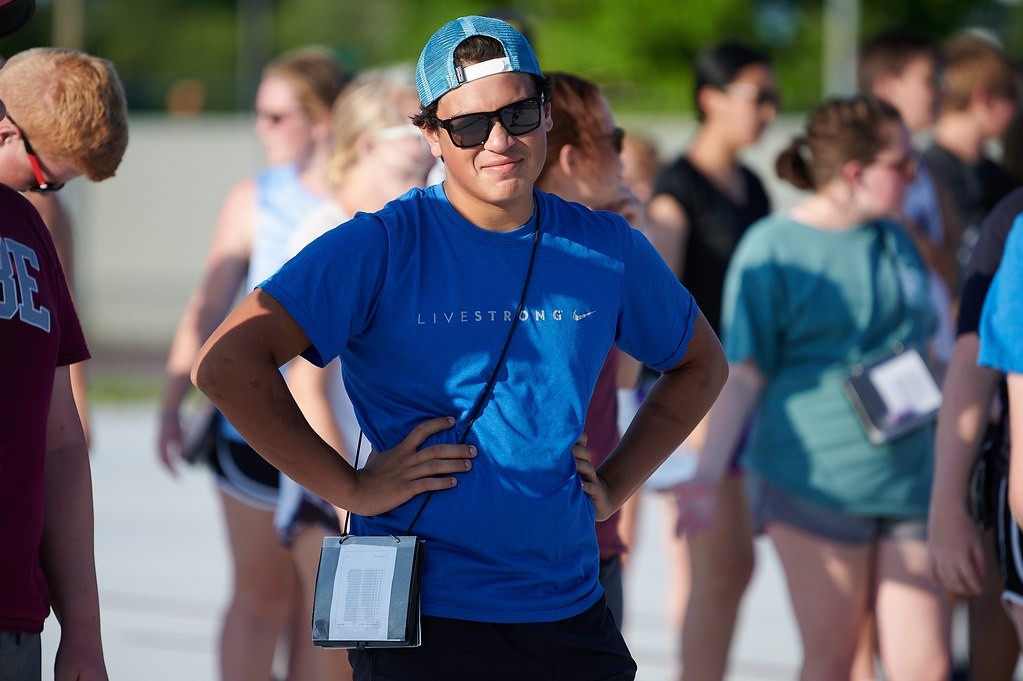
[846,348,945,445]
[311,535,425,650]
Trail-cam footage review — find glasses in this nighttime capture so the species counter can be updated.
[594,127,625,155]
[864,148,921,183]
[6,114,65,194]
[428,91,544,148]
[722,87,778,108]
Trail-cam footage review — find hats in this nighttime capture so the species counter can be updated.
[415,15,545,112]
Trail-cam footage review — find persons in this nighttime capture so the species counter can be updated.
[192,15,729,681]
[0,47,129,195]
[903,25,1023,278]
[1,183,109,680]
[644,43,780,681]
[533,70,626,632]
[653,95,956,681]
[287,61,445,538]
[856,30,964,321]
[981,209,1023,680]
[154,44,355,680]
[925,178,1022,678]
